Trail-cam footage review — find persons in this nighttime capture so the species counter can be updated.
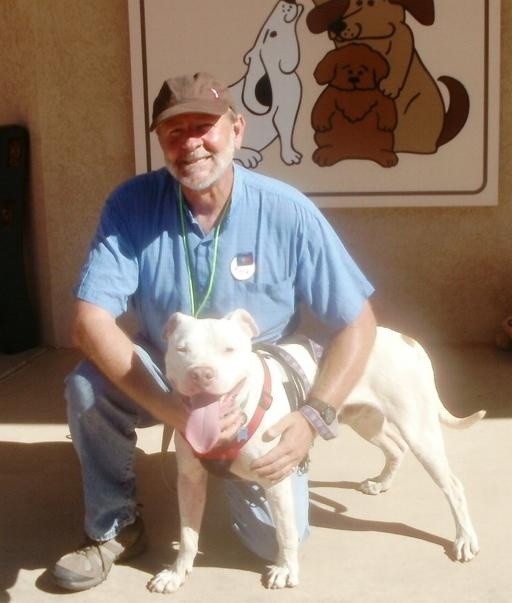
[48,67,380,595]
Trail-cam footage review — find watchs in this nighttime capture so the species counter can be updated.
[307,394,337,426]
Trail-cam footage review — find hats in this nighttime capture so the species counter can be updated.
[149,73,236,131]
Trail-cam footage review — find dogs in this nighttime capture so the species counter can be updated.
[146,306,487,596]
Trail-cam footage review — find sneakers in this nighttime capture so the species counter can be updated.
[52,517,146,590]
